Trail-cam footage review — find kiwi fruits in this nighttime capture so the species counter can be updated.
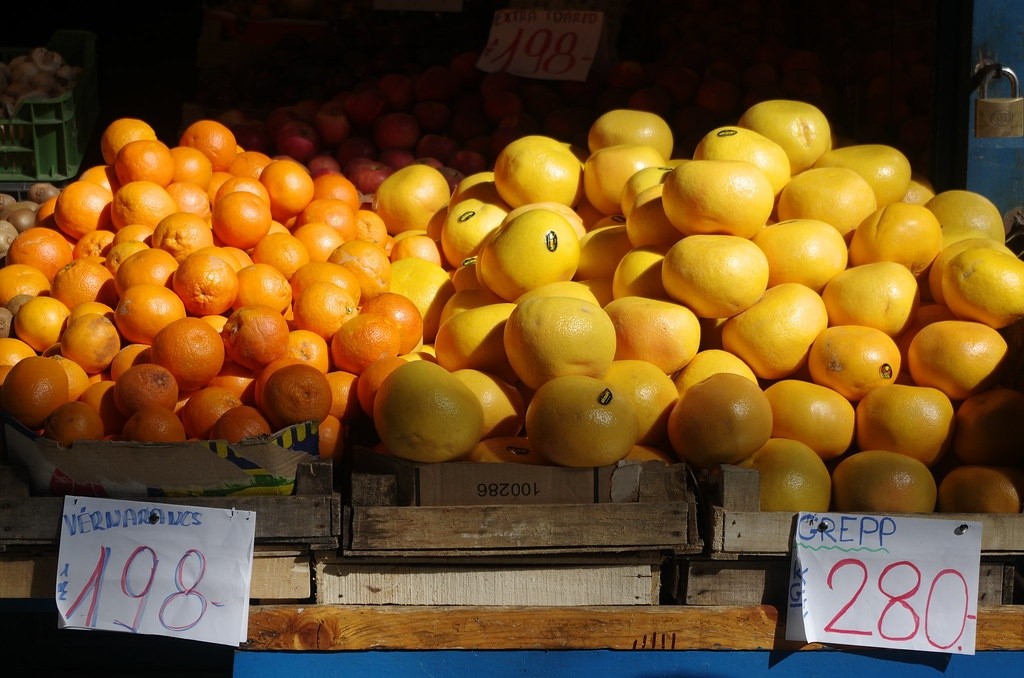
[5,294,35,317]
[6,209,39,234]
[0,194,16,206]
[0,306,15,338]
[28,183,58,204]
[0,219,19,261]
[0,201,41,222]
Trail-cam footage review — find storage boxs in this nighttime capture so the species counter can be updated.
[0,30,99,182]
[0,405,1024,610]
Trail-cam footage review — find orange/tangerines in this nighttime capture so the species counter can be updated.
[0,117,448,466]
[356,98,1024,519]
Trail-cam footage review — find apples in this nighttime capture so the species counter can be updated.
[221,0,944,191]
